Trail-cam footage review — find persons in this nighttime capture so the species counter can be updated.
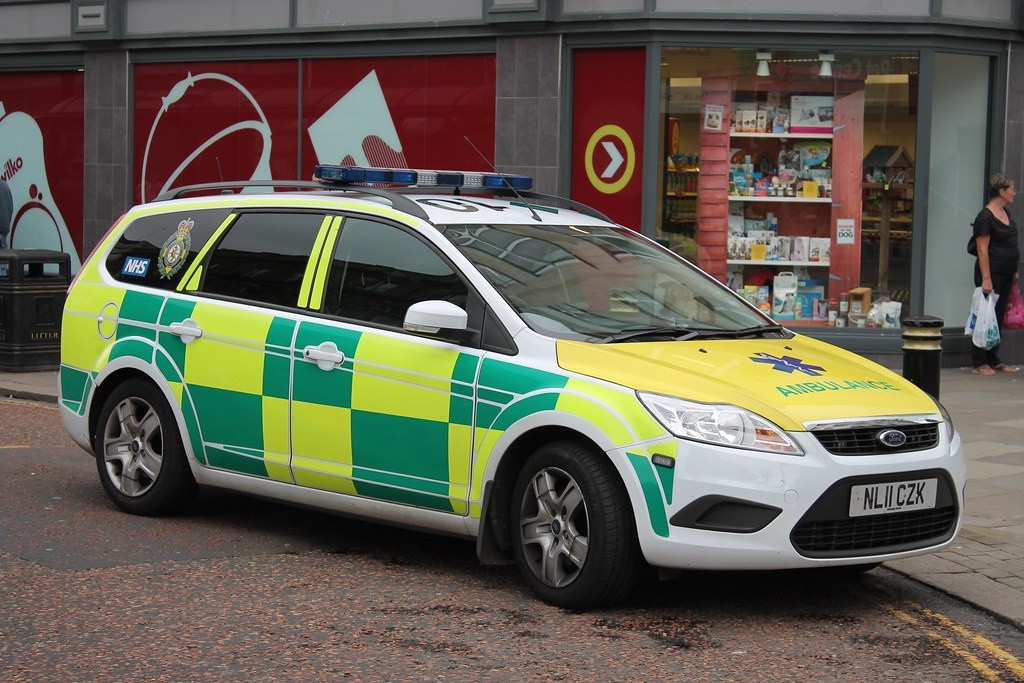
[0,178,14,277]
[970,173,1021,376]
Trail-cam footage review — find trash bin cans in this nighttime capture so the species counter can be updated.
[0,248,72,373]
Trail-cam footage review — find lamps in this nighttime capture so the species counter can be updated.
[754,49,772,77]
[817,50,834,77]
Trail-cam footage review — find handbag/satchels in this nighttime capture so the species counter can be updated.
[973,288,1002,350]
[967,236,977,256]
[964,286,982,336]
[1002,282,1024,330]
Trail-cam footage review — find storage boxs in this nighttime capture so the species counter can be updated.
[729,139,832,198]
[731,96,834,133]
[737,283,871,330]
[729,202,831,263]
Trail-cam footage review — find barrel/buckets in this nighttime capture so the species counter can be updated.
[848,311,868,328]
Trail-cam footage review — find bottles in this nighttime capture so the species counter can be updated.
[828,292,849,328]
[664,172,698,219]
[765,212,777,236]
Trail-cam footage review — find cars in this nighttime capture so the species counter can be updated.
[57,163,969,614]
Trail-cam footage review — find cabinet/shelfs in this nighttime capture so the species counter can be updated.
[728,132,832,326]
[665,168,699,224]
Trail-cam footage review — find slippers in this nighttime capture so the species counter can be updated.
[994,364,1014,373]
[971,365,996,375]
